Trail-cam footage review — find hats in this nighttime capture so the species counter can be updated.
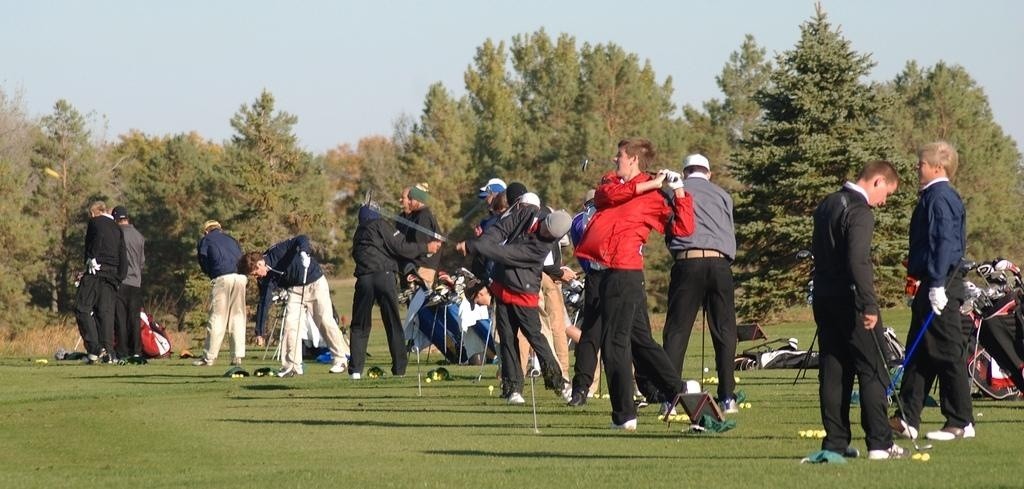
[359,206,379,224]
[112,205,127,219]
[682,154,710,172]
[465,279,485,310]
[478,178,540,209]
[584,189,596,204]
[408,182,429,203]
[545,209,572,239]
[202,219,221,234]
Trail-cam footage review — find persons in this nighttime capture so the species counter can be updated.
[658,154,738,415]
[886,139,976,442]
[71,199,145,366]
[349,177,604,406]
[568,134,704,431]
[193,219,250,368]
[807,158,913,461]
[240,235,350,377]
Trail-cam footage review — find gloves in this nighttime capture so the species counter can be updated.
[300,251,311,268]
[86,258,101,276]
[929,286,949,316]
[662,169,684,190]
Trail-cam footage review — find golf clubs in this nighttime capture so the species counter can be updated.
[330,189,458,250]
[66,306,94,356]
[562,268,729,411]
[581,158,680,182]
[851,283,933,453]
[260,264,308,379]
[397,274,504,383]
[888,256,962,404]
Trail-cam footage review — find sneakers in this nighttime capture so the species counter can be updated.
[611,417,637,430]
[867,441,911,460]
[718,398,738,413]
[685,380,701,393]
[660,401,676,415]
[569,392,586,406]
[329,362,345,373]
[280,361,303,375]
[822,448,859,457]
[192,358,213,366]
[926,422,975,440]
[87,348,143,364]
[508,391,525,404]
[888,416,918,440]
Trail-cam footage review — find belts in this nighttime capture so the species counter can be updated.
[675,249,725,260]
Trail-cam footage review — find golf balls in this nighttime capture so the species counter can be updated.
[223,364,283,379]
[795,426,827,440]
[648,361,752,423]
[908,447,931,464]
[424,363,452,384]
[365,363,389,383]
